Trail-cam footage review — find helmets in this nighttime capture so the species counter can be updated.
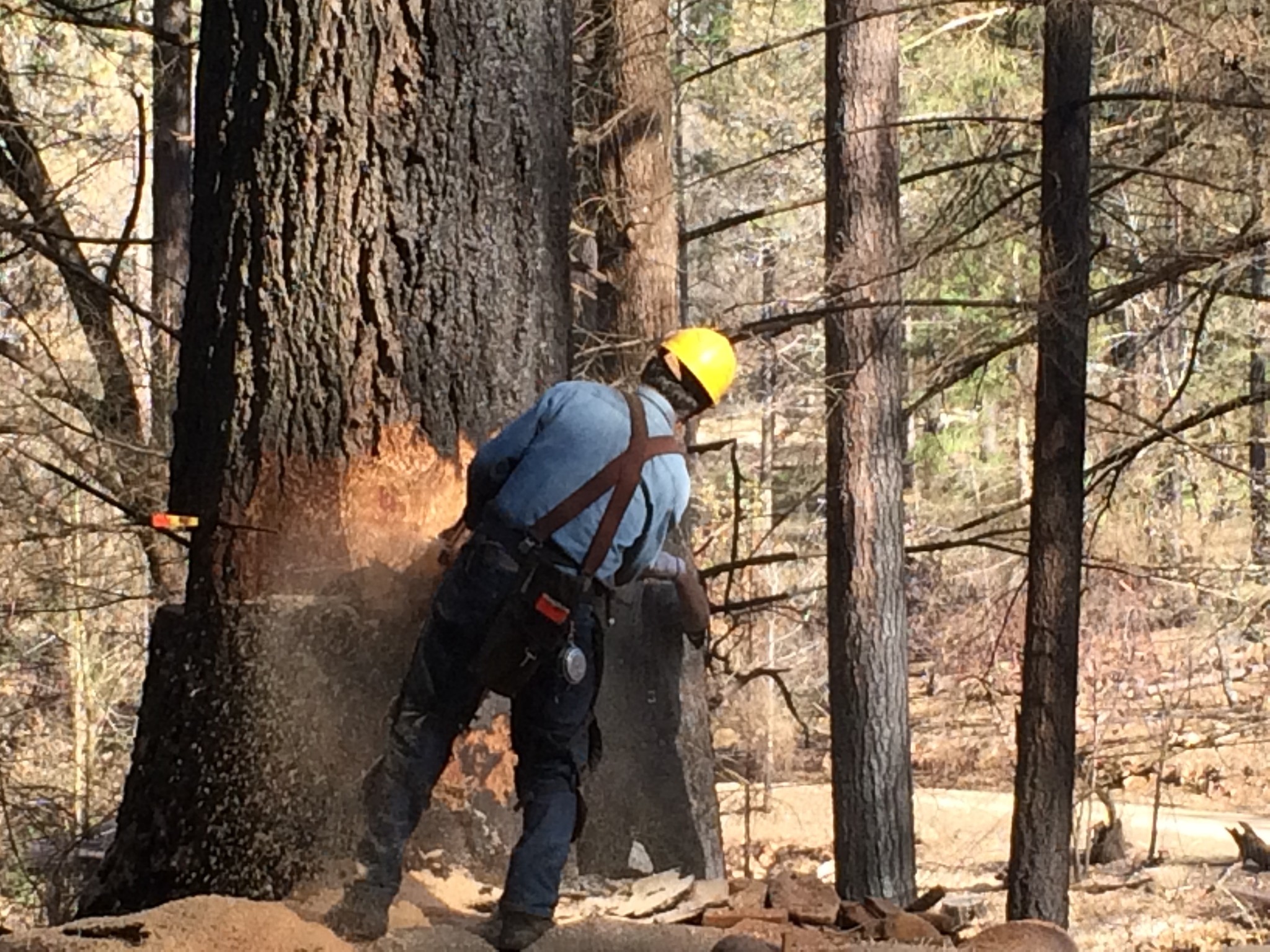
[661,327,737,407]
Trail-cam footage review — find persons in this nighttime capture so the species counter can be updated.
[326,329,739,951]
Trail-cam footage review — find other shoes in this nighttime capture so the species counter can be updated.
[486,911,554,952]
[324,880,395,942]
